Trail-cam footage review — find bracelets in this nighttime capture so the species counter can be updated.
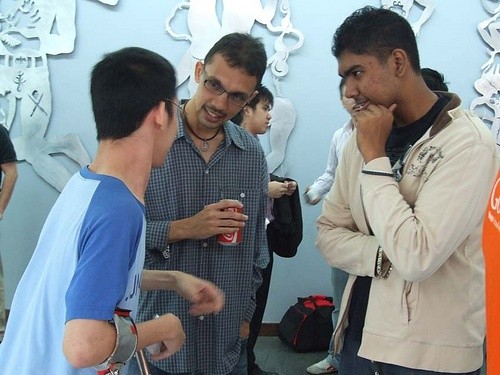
[377,246,392,280]
[362,169,395,180]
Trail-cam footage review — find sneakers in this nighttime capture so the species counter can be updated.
[306,359,337,374]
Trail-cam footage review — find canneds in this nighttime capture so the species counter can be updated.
[216,199,243,246]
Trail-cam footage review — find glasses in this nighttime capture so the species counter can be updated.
[202,65,249,107]
[162,97,186,119]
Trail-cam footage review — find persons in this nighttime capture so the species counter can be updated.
[0,48,225,375]
[230,85,297,375]
[140,32,271,375]
[306,6,500,375]
[0,122,19,342]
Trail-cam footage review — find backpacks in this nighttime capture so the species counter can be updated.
[276,295,335,353]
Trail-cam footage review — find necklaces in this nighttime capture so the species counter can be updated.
[183,98,222,151]
